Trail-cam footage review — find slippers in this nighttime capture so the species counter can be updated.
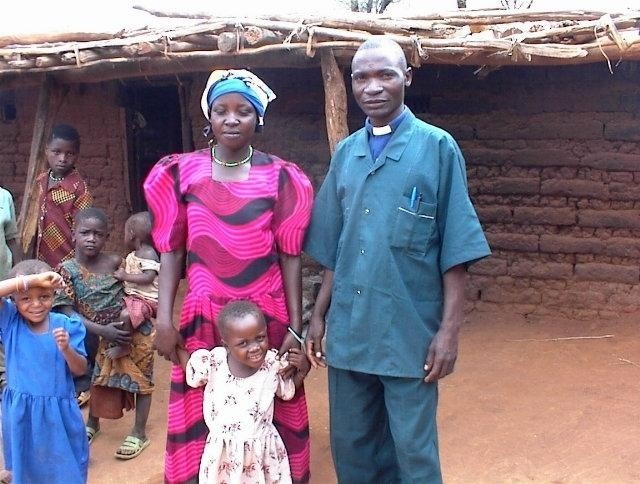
[85,425,101,448]
[114,434,151,459]
[77,389,91,408]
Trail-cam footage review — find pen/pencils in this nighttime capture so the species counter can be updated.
[410,186,423,213]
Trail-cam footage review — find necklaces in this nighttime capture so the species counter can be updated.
[50,170,67,182]
[212,142,253,166]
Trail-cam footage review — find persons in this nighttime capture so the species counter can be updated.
[148,301,312,484]
[102,210,160,360]
[301,33,494,481]
[50,207,156,461]
[0,186,24,483]
[1,260,90,484]
[20,123,95,270]
[140,69,316,483]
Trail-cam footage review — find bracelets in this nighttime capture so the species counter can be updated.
[15,275,20,293]
[23,275,28,292]
[287,325,302,345]
